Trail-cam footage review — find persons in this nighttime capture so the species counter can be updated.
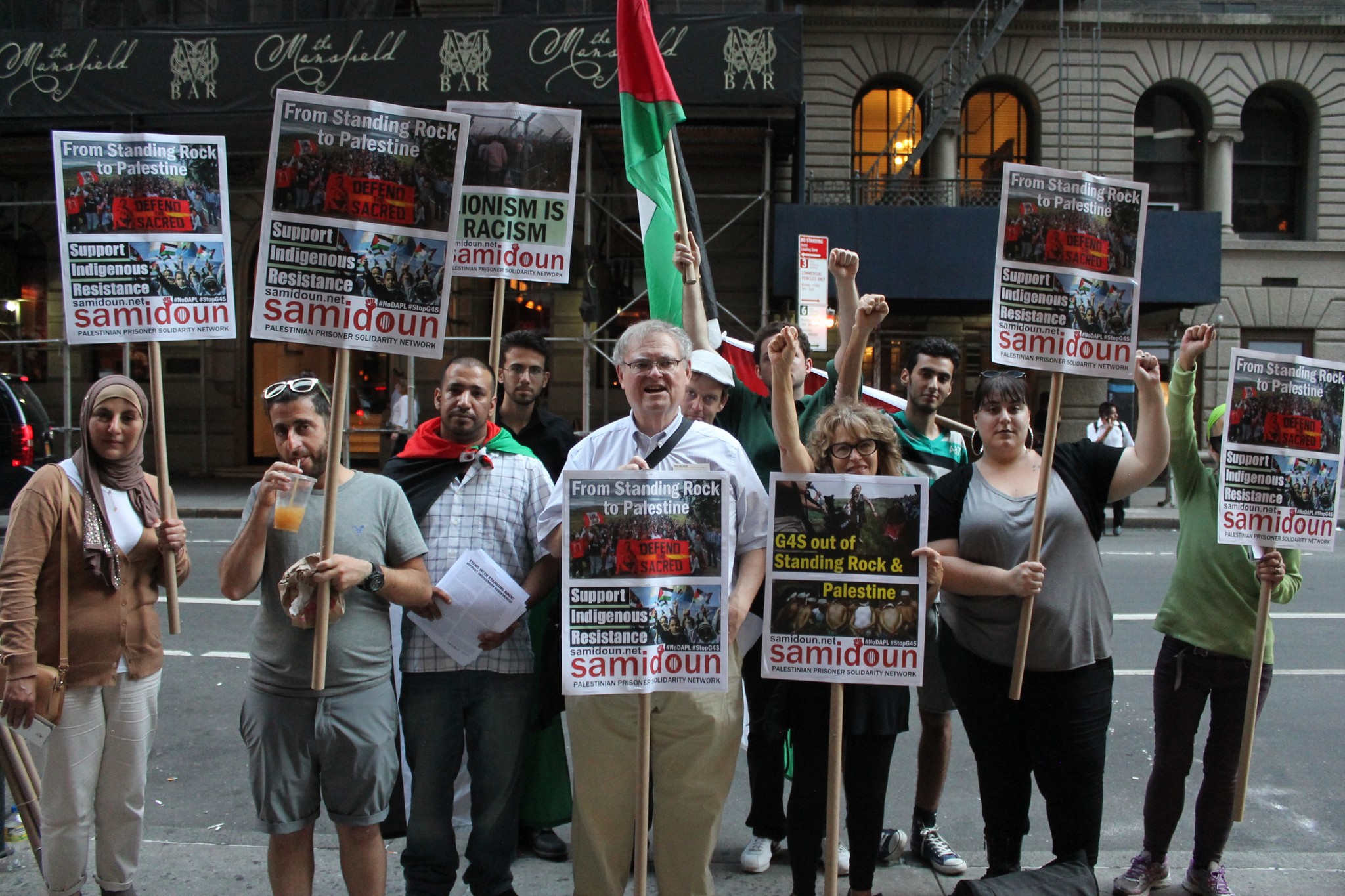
[67,137,1344,646]
[0,232,1302,896]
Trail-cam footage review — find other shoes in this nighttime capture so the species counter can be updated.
[1114,525,1121,537]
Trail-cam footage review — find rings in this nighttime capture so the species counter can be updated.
[1275,567,1280,575]
[1278,560,1281,568]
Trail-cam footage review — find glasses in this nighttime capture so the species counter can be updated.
[264,377,332,414]
[1210,435,1222,453]
[620,359,685,377]
[502,365,547,378]
[826,438,879,460]
[980,369,1028,387]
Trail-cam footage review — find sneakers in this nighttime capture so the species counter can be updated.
[819,836,850,876]
[879,828,908,861]
[1113,851,1171,896]
[524,825,568,859]
[1182,856,1236,896]
[739,830,781,873]
[910,818,967,876]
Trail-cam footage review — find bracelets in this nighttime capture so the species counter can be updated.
[174,547,183,556]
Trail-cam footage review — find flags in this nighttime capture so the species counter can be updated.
[615,0,909,416]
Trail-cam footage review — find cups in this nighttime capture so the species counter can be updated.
[273,471,317,534]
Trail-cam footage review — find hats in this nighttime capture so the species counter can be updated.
[93,385,142,418]
[690,349,735,388]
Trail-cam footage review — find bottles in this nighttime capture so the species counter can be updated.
[4,805,27,871]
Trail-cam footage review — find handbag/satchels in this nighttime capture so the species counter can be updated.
[0,660,66,724]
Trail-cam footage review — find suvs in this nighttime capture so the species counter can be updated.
[1,372,56,515]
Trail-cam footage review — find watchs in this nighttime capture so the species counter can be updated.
[358,560,386,593]
